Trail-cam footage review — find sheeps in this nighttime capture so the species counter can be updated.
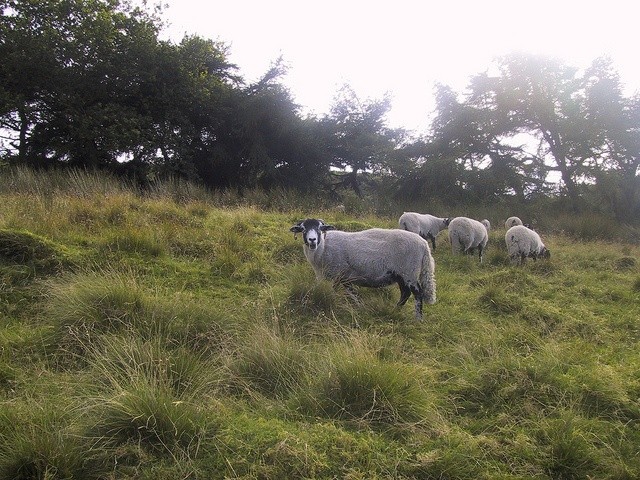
[447,216,491,263]
[504,216,530,233]
[503,223,551,267]
[289,218,437,325]
[399,211,454,251]
[481,218,492,232]
